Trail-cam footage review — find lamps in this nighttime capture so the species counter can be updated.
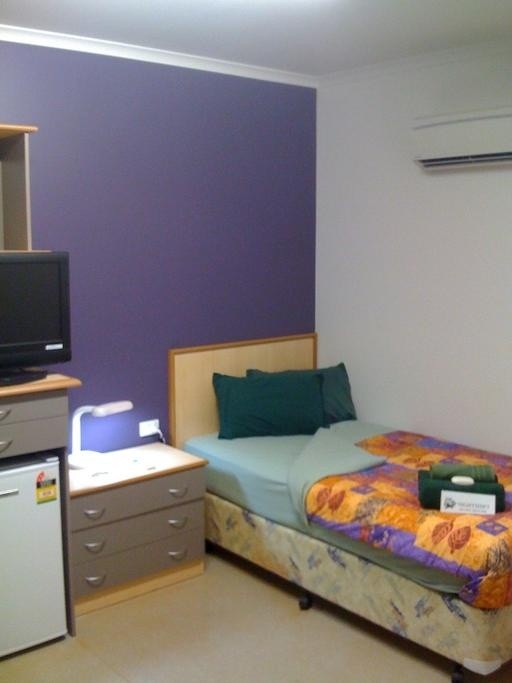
[70,399,134,468]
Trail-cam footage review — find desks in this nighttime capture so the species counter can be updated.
[0,121,83,648]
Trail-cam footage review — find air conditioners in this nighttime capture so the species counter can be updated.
[409,105,512,172]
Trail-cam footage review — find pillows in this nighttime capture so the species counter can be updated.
[211,371,326,440]
[245,361,357,423]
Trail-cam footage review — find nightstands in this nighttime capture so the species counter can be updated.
[68,441,209,618]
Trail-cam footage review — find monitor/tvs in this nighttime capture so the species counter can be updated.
[0,250,72,389]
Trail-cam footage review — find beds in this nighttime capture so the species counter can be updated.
[167,332,511,677]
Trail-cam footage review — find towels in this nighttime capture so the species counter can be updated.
[430,464,495,482]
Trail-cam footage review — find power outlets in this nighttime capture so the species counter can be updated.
[138,419,160,438]
